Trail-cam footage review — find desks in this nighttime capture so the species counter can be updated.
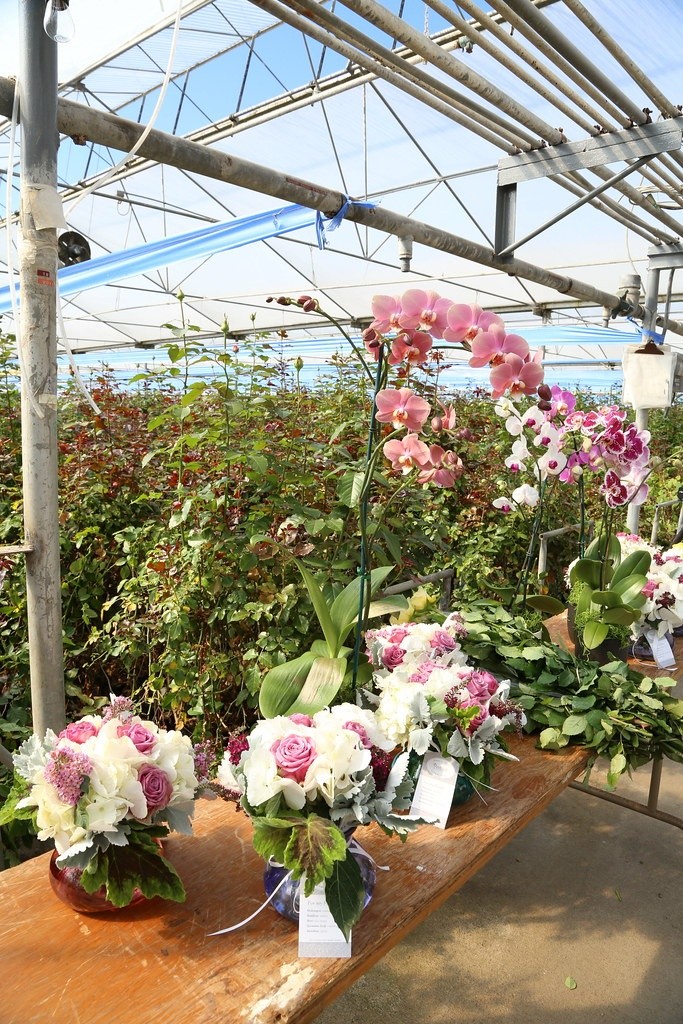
[0,610,683,1024]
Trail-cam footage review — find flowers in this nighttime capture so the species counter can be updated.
[0,287,683,945]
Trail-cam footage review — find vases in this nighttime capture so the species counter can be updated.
[49,837,164,913]
[574,629,629,671]
[567,601,576,645]
[263,827,378,928]
[626,631,674,660]
[406,750,474,808]
